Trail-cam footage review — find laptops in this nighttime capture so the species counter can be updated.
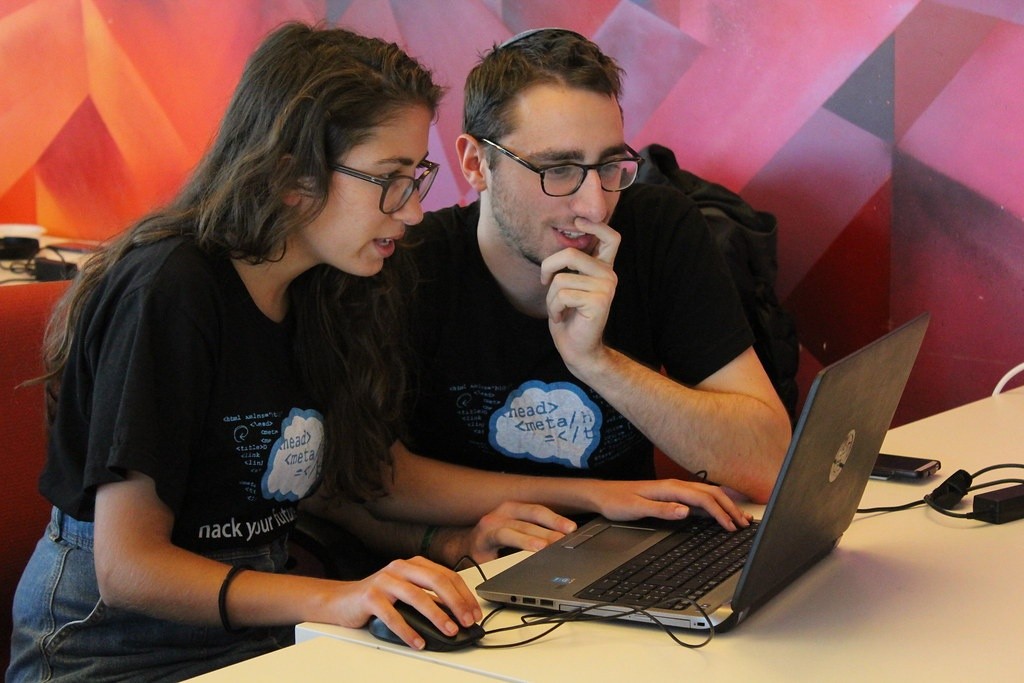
[474,311,933,633]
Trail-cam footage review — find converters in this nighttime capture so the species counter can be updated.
[973,483,1024,525]
[34,257,78,282]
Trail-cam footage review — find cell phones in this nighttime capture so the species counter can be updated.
[872,453,941,480]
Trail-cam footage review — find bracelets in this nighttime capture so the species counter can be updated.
[421,524,440,558]
[218,563,257,635]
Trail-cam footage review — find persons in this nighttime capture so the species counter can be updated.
[5,23,753,683]
[296,27,791,570]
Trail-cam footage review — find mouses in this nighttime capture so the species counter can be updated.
[368,599,485,651]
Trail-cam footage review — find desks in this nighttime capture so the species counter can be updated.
[179,385,1024,683]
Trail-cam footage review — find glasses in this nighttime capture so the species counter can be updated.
[330,160,439,214]
[483,137,646,197]
